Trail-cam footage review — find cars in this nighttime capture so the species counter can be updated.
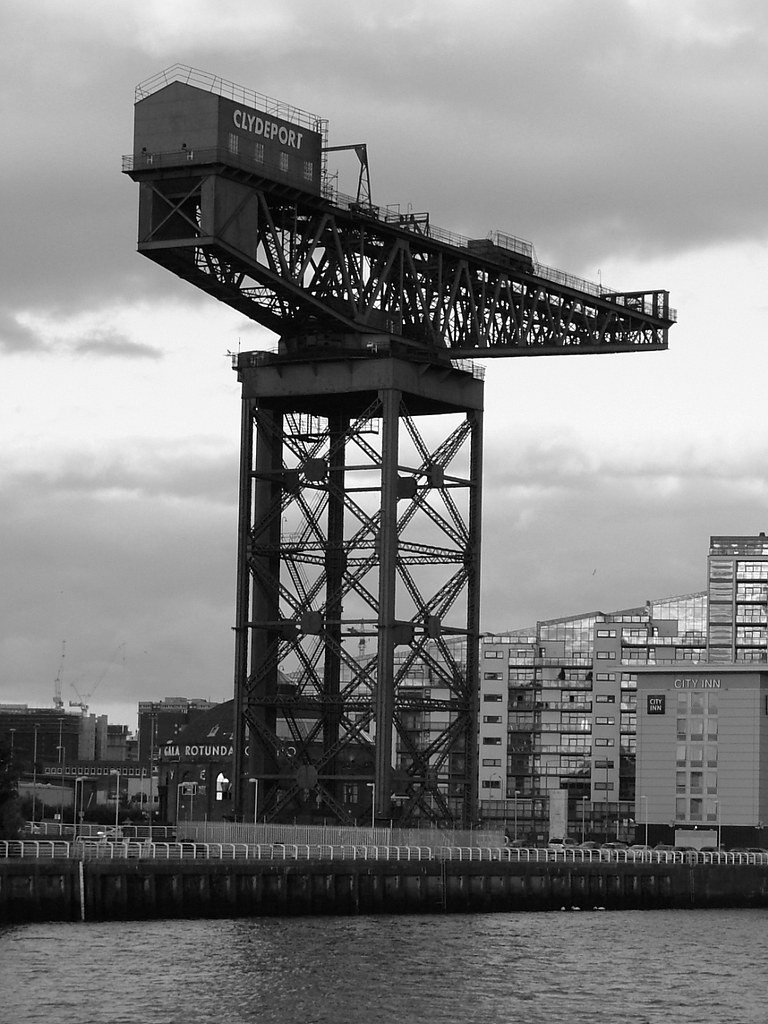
[726,847,768,864]
[628,844,654,861]
[650,845,674,862]
[575,841,602,859]
[671,847,698,863]
[697,846,729,865]
[600,843,629,860]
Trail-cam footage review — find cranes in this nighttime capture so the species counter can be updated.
[51,637,96,716]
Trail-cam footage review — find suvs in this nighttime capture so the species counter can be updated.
[548,838,578,857]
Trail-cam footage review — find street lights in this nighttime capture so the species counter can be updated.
[73,776,88,844]
[56,745,64,834]
[514,791,521,842]
[174,781,198,828]
[249,778,258,826]
[57,716,66,764]
[367,783,375,830]
[641,795,648,852]
[110,769,119,846]
[32,722,40,824]
[713,801,721,852]
[10,728,17,767]
[582,796,589,843]
